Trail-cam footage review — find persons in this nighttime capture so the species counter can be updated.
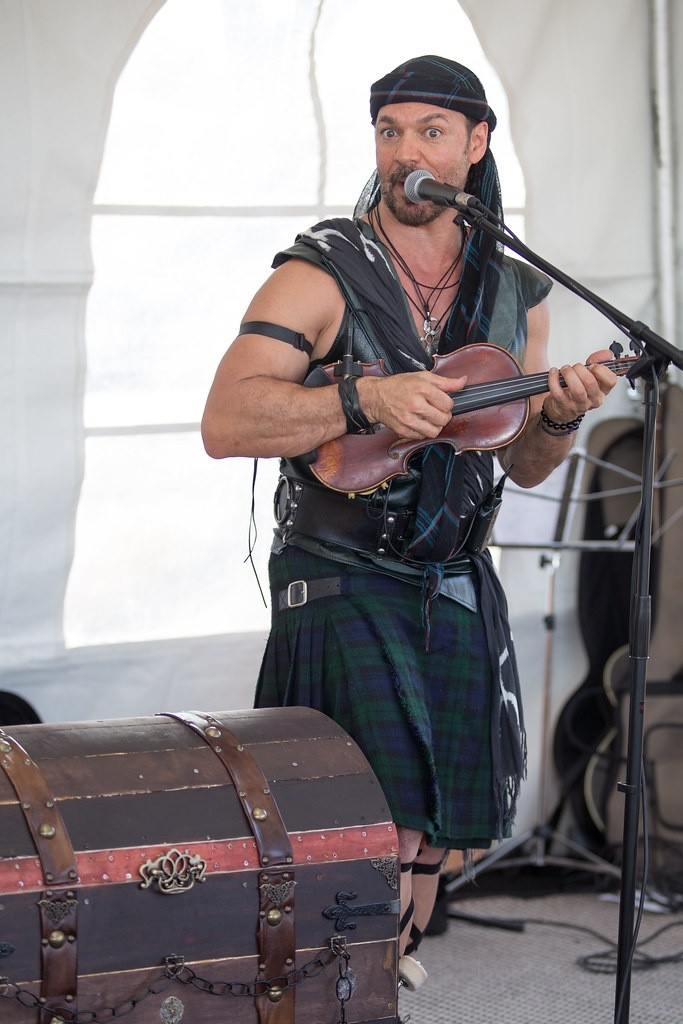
[199,56,618,958]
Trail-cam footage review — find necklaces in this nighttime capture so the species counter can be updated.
[365,204,469,357]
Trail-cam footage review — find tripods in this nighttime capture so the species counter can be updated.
[444,445,683,914]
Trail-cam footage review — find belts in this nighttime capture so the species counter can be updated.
[272,475,471,558]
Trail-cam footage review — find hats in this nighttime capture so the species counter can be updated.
[370,54,498,132]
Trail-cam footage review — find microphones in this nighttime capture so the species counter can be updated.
[404,170,482,209]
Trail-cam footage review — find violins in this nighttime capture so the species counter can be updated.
[299,336,675,498]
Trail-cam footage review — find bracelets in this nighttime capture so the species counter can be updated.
[235,320,314,357]
[534,407,584,429]
[338,375,373,438]
